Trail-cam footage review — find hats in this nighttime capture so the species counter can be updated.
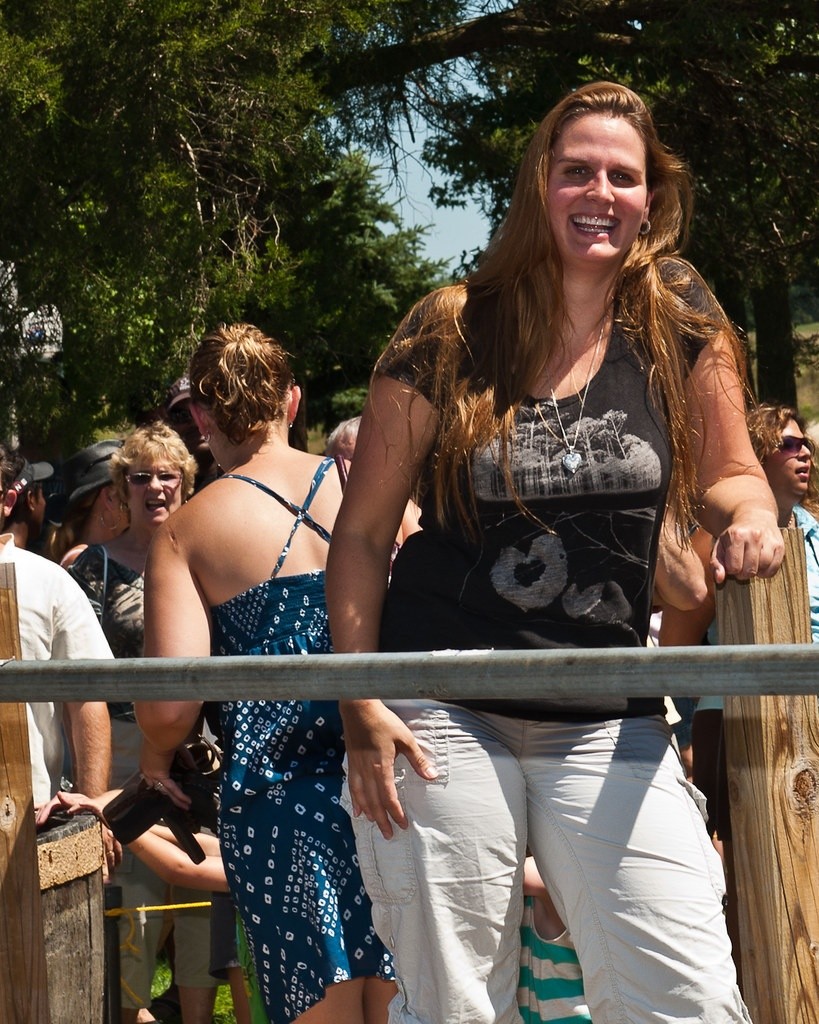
[11,458,54,494]
[59,438,125,501]
[165,377,192,409]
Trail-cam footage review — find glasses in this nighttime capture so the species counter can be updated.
[124,472,182,488]
[777,436,809,454]
[168,409,194,426]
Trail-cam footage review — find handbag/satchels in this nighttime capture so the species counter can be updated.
[101,733,224,863]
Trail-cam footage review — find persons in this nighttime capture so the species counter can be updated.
[325,83,785,1024]
[0,323,819,1024]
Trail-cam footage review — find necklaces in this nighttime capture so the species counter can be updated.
[541,296,614,474]
[786,513,794,528]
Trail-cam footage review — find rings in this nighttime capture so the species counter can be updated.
[106,851,112,855]
[153,782,164,791]
